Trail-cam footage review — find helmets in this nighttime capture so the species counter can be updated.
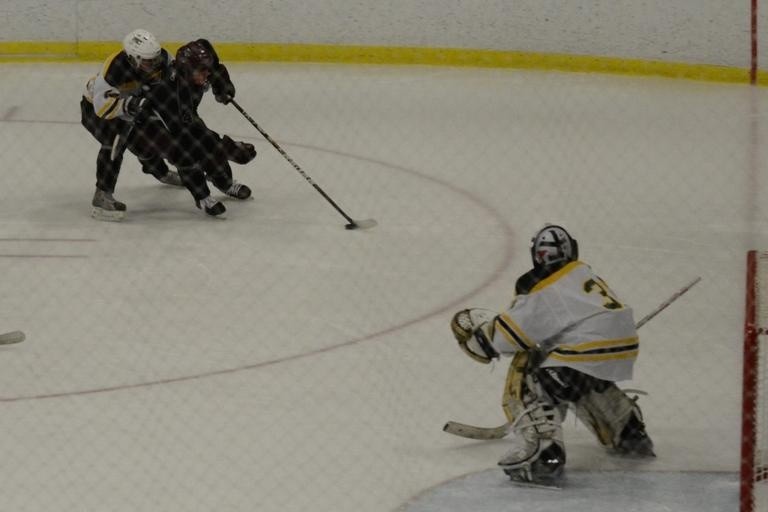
[176,41,214,84]
[124,29,161,69]
[529,222,580,279]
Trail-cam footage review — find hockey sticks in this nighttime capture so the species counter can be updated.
[0,331,23,346]
[228,94,379,229]
[111,90,161,161]
[443,275,700,440]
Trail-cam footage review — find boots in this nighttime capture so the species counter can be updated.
[196,183,251,215]
[160,170,182,186]
[91,187,127,211]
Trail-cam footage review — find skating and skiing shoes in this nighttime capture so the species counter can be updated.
[497,435,566,481]
[615,409,657,459]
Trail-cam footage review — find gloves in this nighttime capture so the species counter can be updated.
[210,64,235,105]
[222,134,257,165]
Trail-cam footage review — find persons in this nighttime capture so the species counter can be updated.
[452,227,652,483]
[78,29,189,211]
[138,39,255,215]
